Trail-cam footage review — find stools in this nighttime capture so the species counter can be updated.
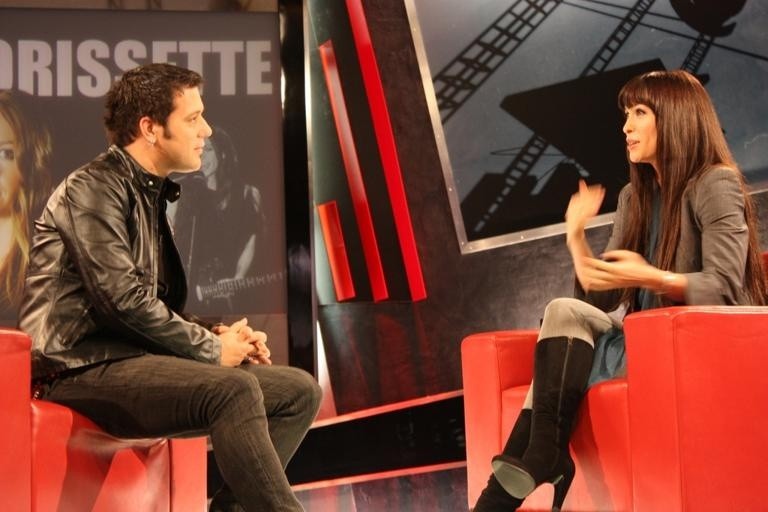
[0,327,210,512]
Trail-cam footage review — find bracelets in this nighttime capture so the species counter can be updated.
[655,270,677,296]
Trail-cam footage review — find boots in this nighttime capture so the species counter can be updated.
[467,404,535,512]
[489,335,597,512]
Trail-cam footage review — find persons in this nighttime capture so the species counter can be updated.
[16,62,323,511]
[0,89,55,327]
[472,70,768,512]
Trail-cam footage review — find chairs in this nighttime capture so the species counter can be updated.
[459,249,768,511]
